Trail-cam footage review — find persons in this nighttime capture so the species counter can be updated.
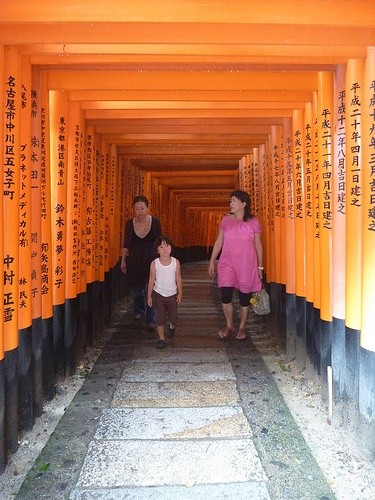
[121,195,162,330]
[209,189,264,340]
[147,236,182,349]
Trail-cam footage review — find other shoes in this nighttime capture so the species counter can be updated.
[166,322,175,338]
[218,326,236,340]
[236,328,247,341]
[156,340,168,349]
[133,313,142,319]
[145,323,155,330]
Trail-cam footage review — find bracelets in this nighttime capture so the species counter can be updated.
[258,266,263,270]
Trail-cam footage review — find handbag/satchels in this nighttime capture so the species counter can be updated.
[250,281,271,315]
